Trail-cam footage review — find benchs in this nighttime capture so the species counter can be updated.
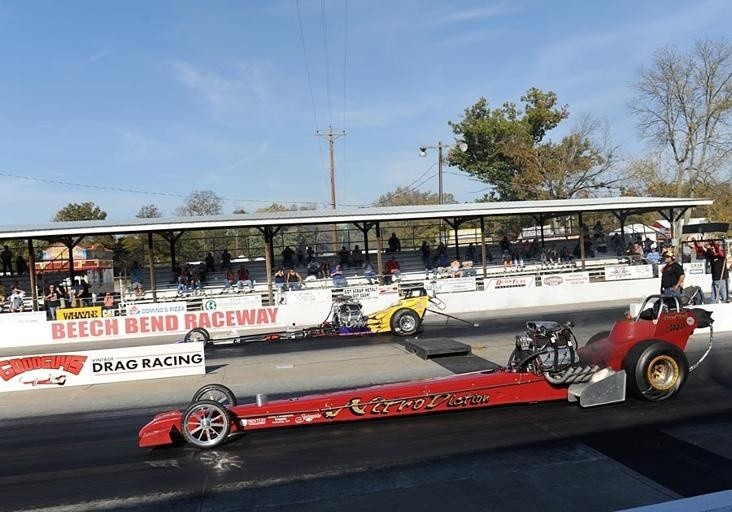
[0,233,660,313]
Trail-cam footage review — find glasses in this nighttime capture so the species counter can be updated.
[662,255,671,258]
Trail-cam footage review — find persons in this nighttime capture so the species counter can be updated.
[129,261,145,296]
[388,233,401,252]
[420,241,477,278]
[661,251,685,300]
[485,244,492,262]
[174,249,253,292]
[385,257,402,277]
[0,245,29,277]
[498,236,573,268]
[700,240,728,301]
[7,280,114,321]
[274,244,376,292]
[573,221,671,265]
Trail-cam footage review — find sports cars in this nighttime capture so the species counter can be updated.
[137,292,714,451]
[176,287,432,349]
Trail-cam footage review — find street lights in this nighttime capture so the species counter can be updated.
[417,139,469,243]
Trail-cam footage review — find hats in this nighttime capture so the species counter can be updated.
[663,251,673,256]
